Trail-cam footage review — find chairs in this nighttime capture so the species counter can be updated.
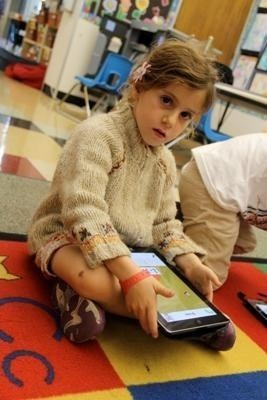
[169,95,235,154]
[56,54,133,117]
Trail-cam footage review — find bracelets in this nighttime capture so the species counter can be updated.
[118,269,149,296]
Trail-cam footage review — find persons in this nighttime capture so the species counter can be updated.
[178,133,266,291]
[25,41,236,352]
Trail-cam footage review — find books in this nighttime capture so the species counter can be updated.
[0,2,56,62]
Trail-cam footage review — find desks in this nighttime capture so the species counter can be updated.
[211,81,267,137]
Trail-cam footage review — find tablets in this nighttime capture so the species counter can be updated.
[129,248,230,337]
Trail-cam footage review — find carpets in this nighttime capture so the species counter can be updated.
[1,230,267,400]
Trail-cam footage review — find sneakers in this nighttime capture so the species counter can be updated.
[50,278,106,344]
[158,319,236,351]
